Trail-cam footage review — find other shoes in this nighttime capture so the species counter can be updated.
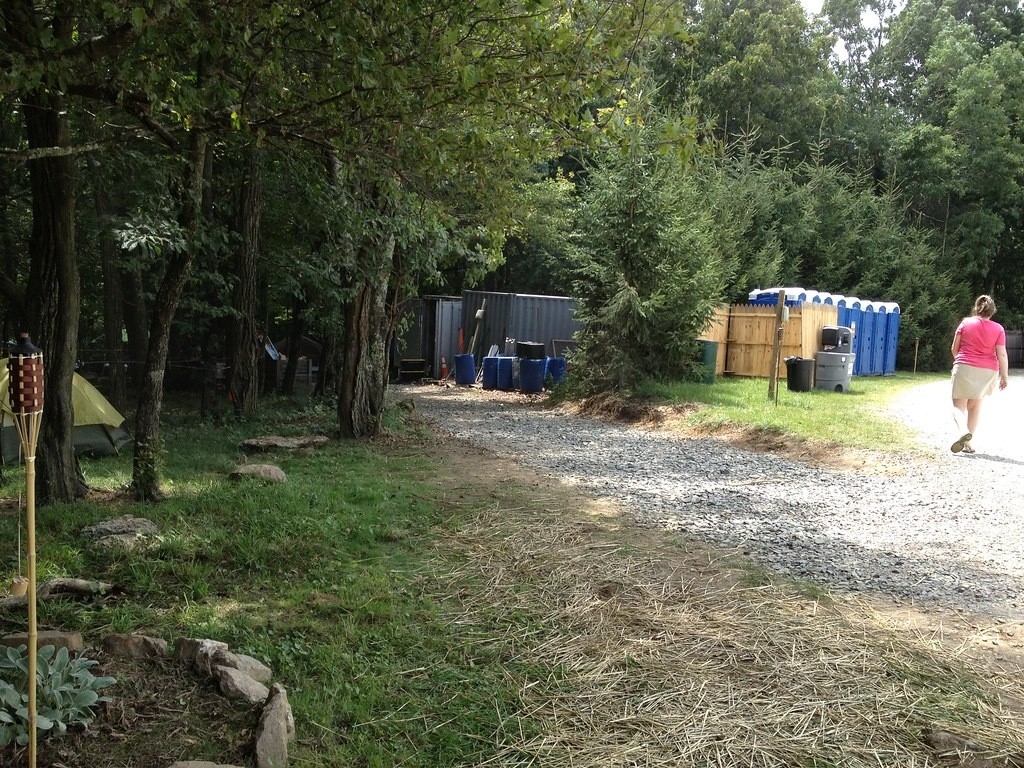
[961,442,975,453]
[951,430,972,453]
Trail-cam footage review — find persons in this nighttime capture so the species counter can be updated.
[950,294,1009,453]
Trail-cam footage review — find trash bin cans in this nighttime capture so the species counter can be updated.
[785,357,816,392]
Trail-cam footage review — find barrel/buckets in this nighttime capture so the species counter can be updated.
[482,357,498,391]
[495,357,516,390]
[544,356,566,386]
[518,358,546,392]
[787,358,816,393]
[455,354,476,385]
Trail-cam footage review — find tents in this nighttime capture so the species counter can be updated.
[0,356,136,467]
